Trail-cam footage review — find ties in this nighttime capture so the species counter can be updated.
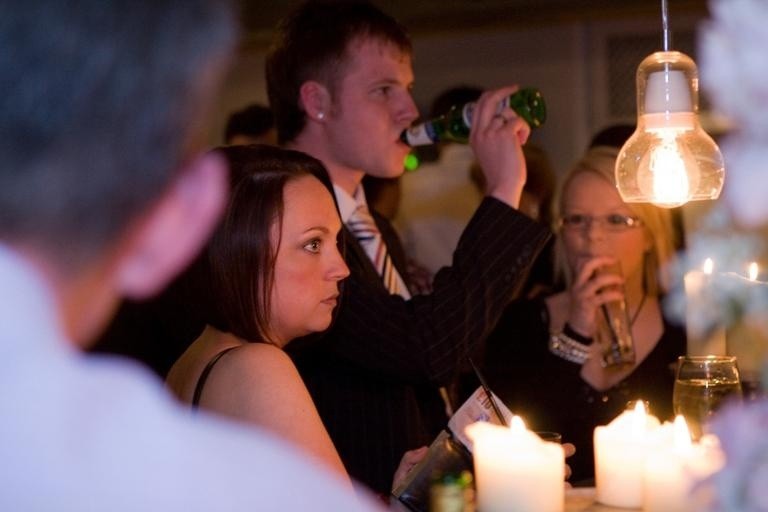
[347,204,409,300]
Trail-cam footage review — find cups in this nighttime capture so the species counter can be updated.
[673,356,743,443]
[587,260,636,370]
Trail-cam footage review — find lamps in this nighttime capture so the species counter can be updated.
[615,0,726,209]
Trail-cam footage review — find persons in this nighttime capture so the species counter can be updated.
[0,0,690,512]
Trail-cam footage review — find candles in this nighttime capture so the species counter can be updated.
[472,259,768,512]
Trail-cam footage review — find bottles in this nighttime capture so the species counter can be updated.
[400,86,547,147]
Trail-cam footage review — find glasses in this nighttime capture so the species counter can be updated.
[557,211,644,242]
